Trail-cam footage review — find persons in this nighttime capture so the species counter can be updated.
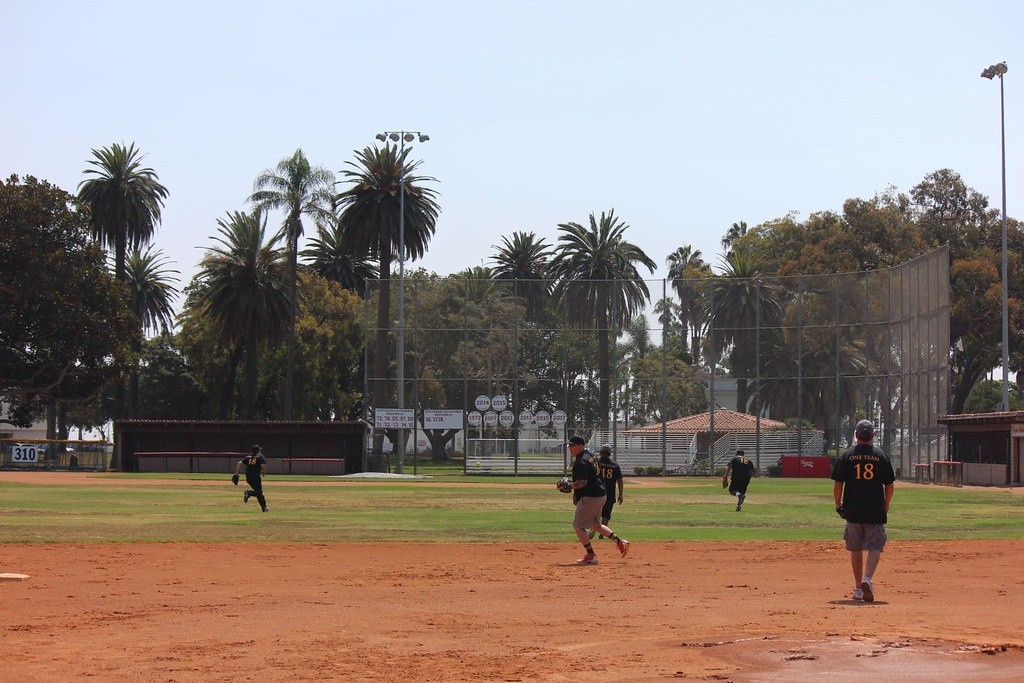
[722,450,754,511]
[231,445,269,513]
[831,420,896,603]
[556,435,630,564]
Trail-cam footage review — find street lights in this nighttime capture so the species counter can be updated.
[375,130,431,475]
[981,59,1009,411]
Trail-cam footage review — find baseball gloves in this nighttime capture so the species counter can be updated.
[722,476,728,489]
[557,477,572,494]
[230,474,240,486]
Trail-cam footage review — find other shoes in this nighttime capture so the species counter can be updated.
[262,508,269,512]
[599,535,603,539]
[588,529,596,539]
[244,490,250,502]
[736,507,741,511]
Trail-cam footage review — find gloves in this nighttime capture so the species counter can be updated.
[836,503,848,519]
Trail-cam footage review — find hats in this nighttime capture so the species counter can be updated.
[856,419,874,437]
[564,437,585,445]
[600,446,611,454]
[735,451,744,455]
[251,445,260,453]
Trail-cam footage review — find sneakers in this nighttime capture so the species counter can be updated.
[852,587,862,599]
[616,540,631,559]
[576,554,599,565]
[860,581,874,602]
